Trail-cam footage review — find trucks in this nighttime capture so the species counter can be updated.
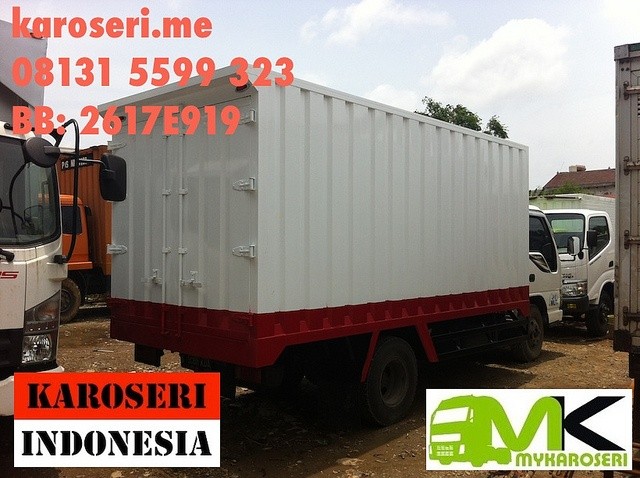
[611,39,640,445]
[56,139,110,326]
[533,178,612,340]
[1,116,128,425]
[97,61,565,428]
